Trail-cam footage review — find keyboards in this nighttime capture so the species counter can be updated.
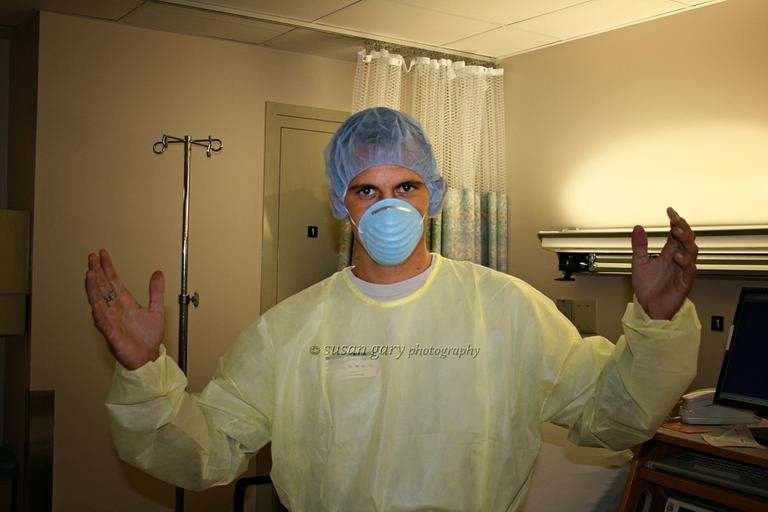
[648,445,768,497]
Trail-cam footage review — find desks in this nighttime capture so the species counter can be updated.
[624,416,768,511]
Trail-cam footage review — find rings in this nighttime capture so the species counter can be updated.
[104,289,116,303]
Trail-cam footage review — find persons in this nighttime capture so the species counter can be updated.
[86,106,702,512]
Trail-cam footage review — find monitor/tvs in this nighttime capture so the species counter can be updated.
[713,287,768,416]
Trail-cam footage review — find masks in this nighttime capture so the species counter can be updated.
[357,197,425,268]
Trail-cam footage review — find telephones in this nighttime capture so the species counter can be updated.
[679,388,753,426]
